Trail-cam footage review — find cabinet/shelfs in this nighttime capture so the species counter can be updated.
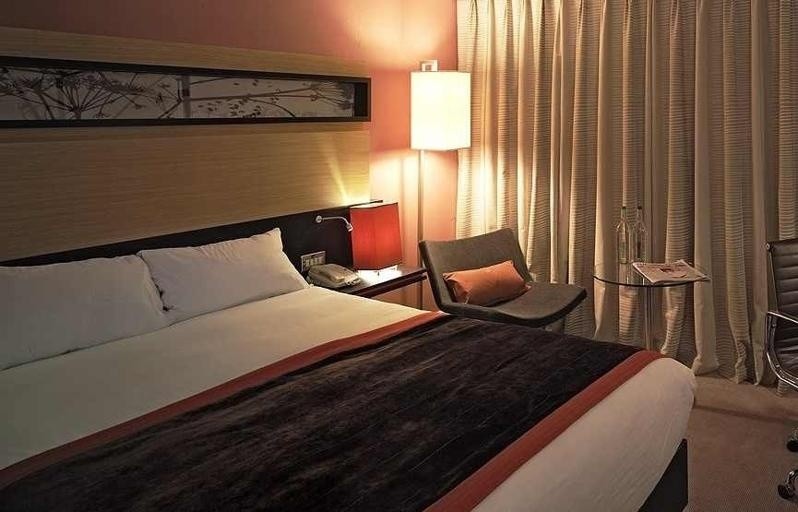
[0,54,375,129]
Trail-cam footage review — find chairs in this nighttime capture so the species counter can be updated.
[415,224,589,329]
[760,234,798,501]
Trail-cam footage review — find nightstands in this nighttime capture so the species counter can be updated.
[314,262,431,312]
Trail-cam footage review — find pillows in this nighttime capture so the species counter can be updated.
[442,258,532,307]
[0,252,172,371]
[134,226,310,327]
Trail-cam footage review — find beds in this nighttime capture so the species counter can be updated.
[0,281,702,512]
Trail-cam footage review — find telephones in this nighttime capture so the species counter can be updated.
[308,263,361,290]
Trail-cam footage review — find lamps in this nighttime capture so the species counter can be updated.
[408,59,476,311]
[312,213,355,233]
[346,202,406,278]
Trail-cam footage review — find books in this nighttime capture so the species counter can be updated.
[633,258,711,285]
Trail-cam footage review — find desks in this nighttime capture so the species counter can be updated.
[587,256,712,352]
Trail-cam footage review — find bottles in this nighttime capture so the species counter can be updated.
[615,206,631,265]
[631,206,646,265]
